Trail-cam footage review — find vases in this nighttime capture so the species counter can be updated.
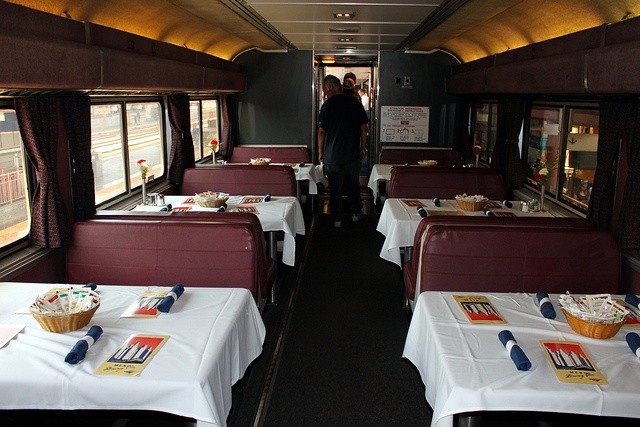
[142,179,146,205]
[540,185,545,212]
[213,151,215,164]
[475,154,479,168]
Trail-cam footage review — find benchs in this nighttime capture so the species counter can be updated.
[390,165,497,198]
[187,165,296,197]
[391,169,505,200]
[381,149,457,165]
[230,147,311,164]
[402,214,593,312]
[65,219,262,308]
[420,225,621,295]
[91,210,277,296]
[181,170,295,196]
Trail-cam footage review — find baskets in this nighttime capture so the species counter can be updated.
[418,160,438,167]
[193,192,228,207]
[562,294,625,339]
[455,196,489,212]
[250,158,272,166]
[30,288,100,333]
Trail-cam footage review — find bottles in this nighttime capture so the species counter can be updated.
[520,201,530,213]
[157,194,165,206]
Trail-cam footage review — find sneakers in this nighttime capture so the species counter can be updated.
[351,214,361,222]
[334,222,341,227]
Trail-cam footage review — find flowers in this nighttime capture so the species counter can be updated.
[529,150,559,186]
[136,159,151,179]
[472,143,482,154]
[207,140,223,151]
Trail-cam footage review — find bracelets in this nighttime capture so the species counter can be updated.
[362,144,368,149]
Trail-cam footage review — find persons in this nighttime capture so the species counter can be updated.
[574,182,589,200]
[316,74,370,229]
[358,88,369,120]
[343,72,357,84]
[343,77,359,101]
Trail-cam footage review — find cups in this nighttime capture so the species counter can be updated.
[216,160,224,165]
[528,199,540,212]
[144,192,158,206]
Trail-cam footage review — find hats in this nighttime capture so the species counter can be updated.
[343,81,356,89]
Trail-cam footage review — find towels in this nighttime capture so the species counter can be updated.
[157,284,184,313]
[417,206,427,217]
[299,161,304,167]
[482,208,496,218]
[536,292,556,319]
[160,204,171,211]
[264,193,271,201]
[500,200,512,208]
[432,196,440,207]
[627,333,639,362]
[66,326,103,364]
[625,293,639,309]
[83,283,96,291]
[449,159,455,169]
[217,203,227,212]
[498,330,531,370]
